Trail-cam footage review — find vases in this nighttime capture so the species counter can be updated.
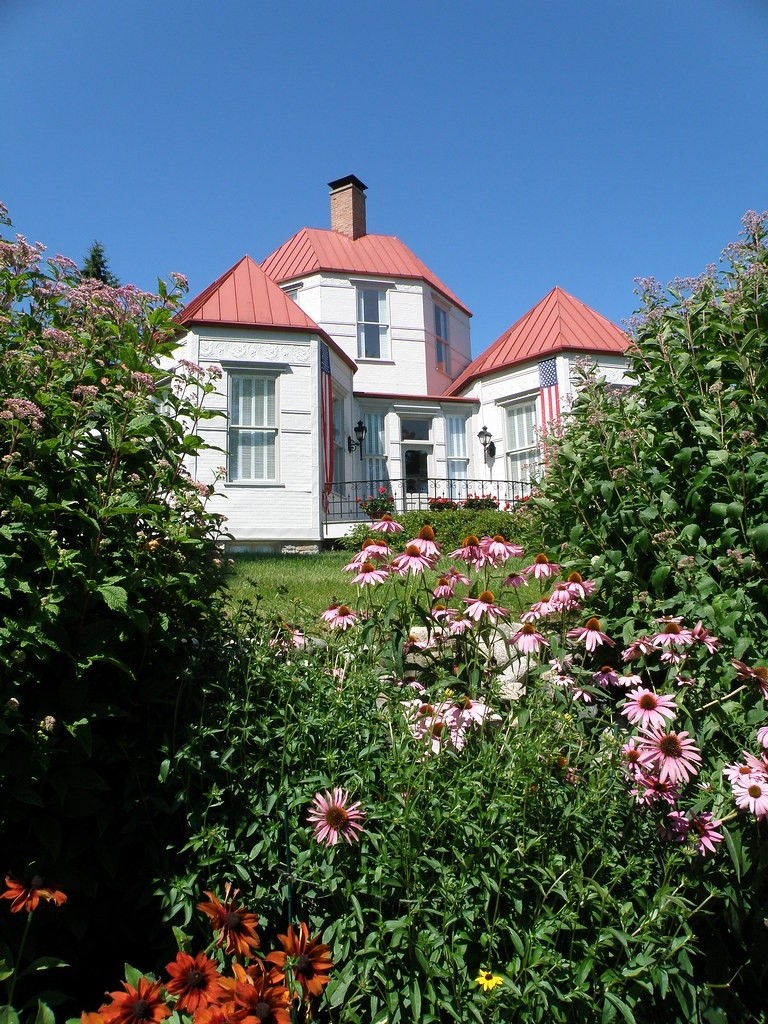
[371,509,385,519]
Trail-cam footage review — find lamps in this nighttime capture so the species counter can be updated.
[348,420,368,461]
[477,426,496,465]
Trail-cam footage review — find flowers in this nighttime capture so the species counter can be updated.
[355,487,396,516]
